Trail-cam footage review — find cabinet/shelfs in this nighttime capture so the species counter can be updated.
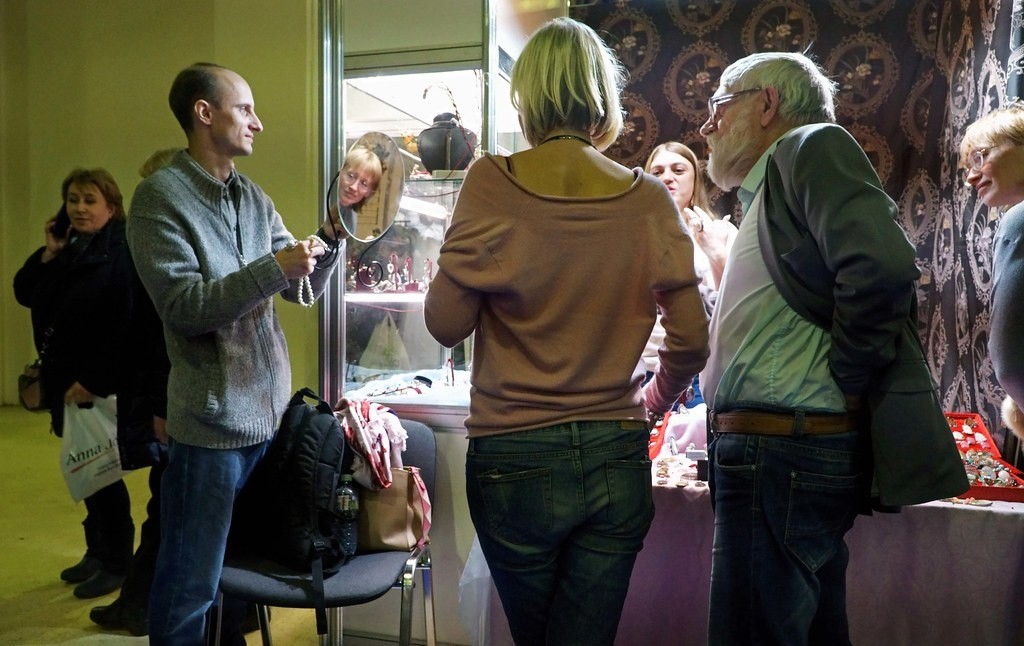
[332,48,535,411]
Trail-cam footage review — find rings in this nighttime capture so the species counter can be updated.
[698,224,705,233]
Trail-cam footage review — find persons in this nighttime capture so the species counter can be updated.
[424,15,710,646]
[956,98,1024,428]
[125,62,349,645]
[338,146,382,233]
[90,265,171,636]
[646,141,739,412]
[700,41,971,646]
[13,168,137,598]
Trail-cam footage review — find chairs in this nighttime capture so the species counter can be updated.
[207,416,438,645]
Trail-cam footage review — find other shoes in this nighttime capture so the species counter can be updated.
[73,568,127,598]
[60,557,102,581]
[89,597,149,635]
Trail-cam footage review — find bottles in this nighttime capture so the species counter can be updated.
[419,112,476,175]
[336,473,358,557]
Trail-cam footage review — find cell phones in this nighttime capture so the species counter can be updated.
[50,203,69,239]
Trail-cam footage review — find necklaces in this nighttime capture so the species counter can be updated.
[539,135,598,150]
[285,234,333,307]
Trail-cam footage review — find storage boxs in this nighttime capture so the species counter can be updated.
[944,413,1024,502]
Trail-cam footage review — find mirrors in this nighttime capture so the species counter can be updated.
[336,132,404,245]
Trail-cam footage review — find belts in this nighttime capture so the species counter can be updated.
[708,410,863,433]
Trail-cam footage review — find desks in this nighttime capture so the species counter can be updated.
[484,460,1024,645]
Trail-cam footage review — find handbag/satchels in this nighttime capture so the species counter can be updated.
[358,466,432,550]
[60,396,130,504]
[361,313,410,372]
[19,362,50,410]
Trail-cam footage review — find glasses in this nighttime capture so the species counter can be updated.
[964,140,1010,174]
[707,87,763,122]
[344,171,373,194]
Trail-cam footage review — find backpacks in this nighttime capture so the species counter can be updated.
[223,387,348,574]
